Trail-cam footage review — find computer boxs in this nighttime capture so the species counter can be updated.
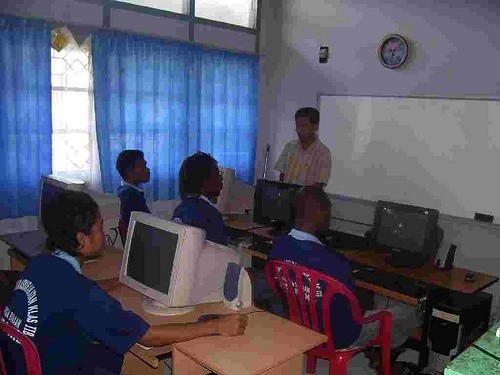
[426,291,493,374]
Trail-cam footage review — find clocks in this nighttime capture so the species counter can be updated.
[378,34,409,69]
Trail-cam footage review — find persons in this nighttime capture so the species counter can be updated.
[171,151,229,246]
[272,107,332,189]
[115,149,151,224]
[270,187,423,375]
[0,187,248,375]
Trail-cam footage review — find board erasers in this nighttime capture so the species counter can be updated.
[474,213,494,223]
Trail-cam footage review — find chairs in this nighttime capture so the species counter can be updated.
[0,306,42,375]
[265,260,393,375]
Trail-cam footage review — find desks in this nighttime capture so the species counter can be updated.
[0,215,499,375]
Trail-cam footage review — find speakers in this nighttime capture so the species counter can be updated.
[222,262,252,310]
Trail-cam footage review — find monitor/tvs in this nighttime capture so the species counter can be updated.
[37,174,120,235]
[119,210,241,316]
[213,168,255,221]
[253,179,304,236]
[371,200,440,268]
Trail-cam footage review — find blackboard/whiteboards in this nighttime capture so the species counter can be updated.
[316,92,500,226]
